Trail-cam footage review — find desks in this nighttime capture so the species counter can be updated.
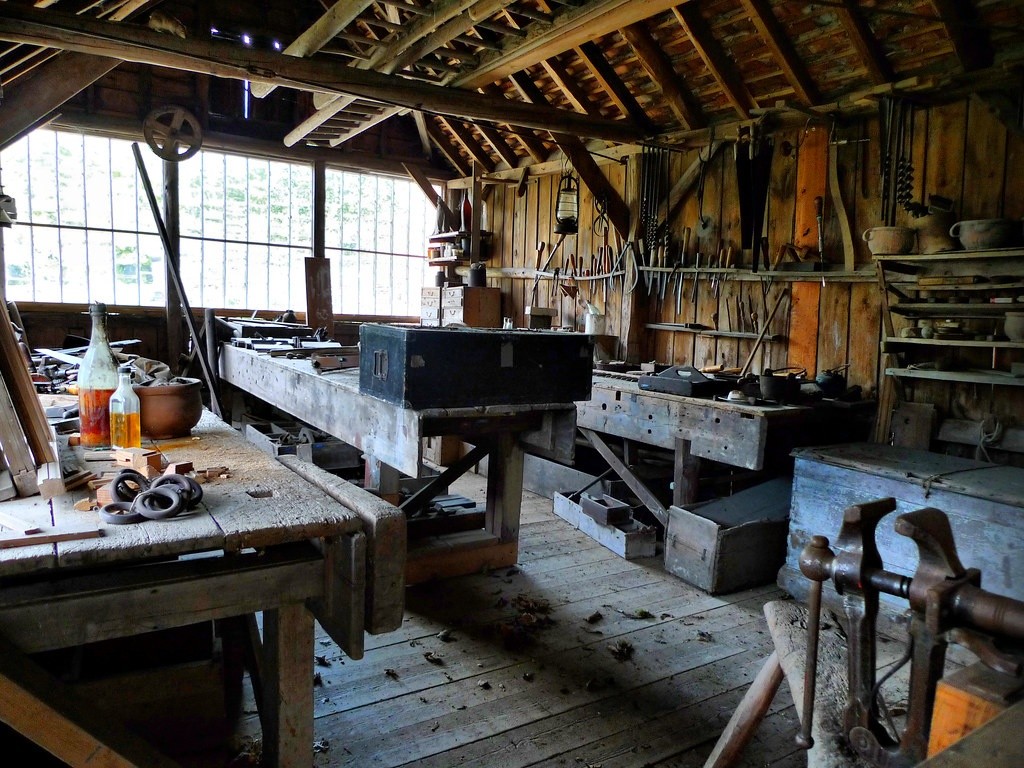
[573,370,877,530]
[218,342,577,581]
[1,413,408,768]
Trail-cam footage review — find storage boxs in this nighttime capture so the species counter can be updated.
[926,662,1024,759]
[357,323,593,410]
[664,495,787,597]
[777,442,1024,668]
[552,489,656,559]
[23,620,235,757]
[239,413,447,502]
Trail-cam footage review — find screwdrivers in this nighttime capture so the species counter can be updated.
[765,244,787,295]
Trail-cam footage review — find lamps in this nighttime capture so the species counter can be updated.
[552,172,580,234]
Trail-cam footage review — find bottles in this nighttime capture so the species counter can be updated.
[110,368,140,452]
[460,190,472,231]
[78,303,121,448]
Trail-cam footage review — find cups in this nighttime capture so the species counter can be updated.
[471,268,486,286]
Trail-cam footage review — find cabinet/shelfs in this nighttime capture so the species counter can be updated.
[428,230,492,265]
[872,245,1024,446]
[420,281,501,328]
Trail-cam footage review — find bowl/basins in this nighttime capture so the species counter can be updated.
[132,376,202,439]
